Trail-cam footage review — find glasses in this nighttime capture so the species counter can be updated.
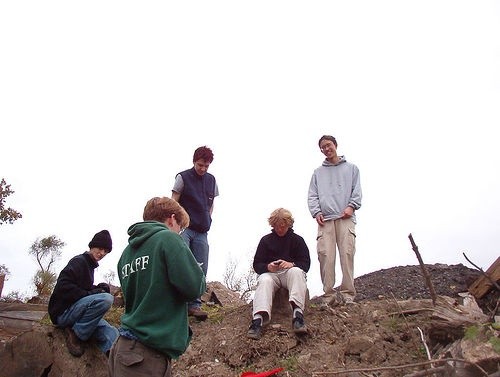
[320,143,334,152]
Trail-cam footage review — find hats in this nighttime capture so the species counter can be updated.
[88,229,113,252]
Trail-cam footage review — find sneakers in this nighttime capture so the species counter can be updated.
[292,318,306,331]
[247,318,261,339]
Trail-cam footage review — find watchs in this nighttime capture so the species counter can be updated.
[292,262,296,266]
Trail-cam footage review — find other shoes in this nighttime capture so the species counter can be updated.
[187,307,209,315]
[66,326,84,355]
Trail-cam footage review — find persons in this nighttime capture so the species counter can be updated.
[247,208,311,339]
[170,146,220,317]
[48,230,121,357]
[308,135,362,310]
[105,196,206,377]
